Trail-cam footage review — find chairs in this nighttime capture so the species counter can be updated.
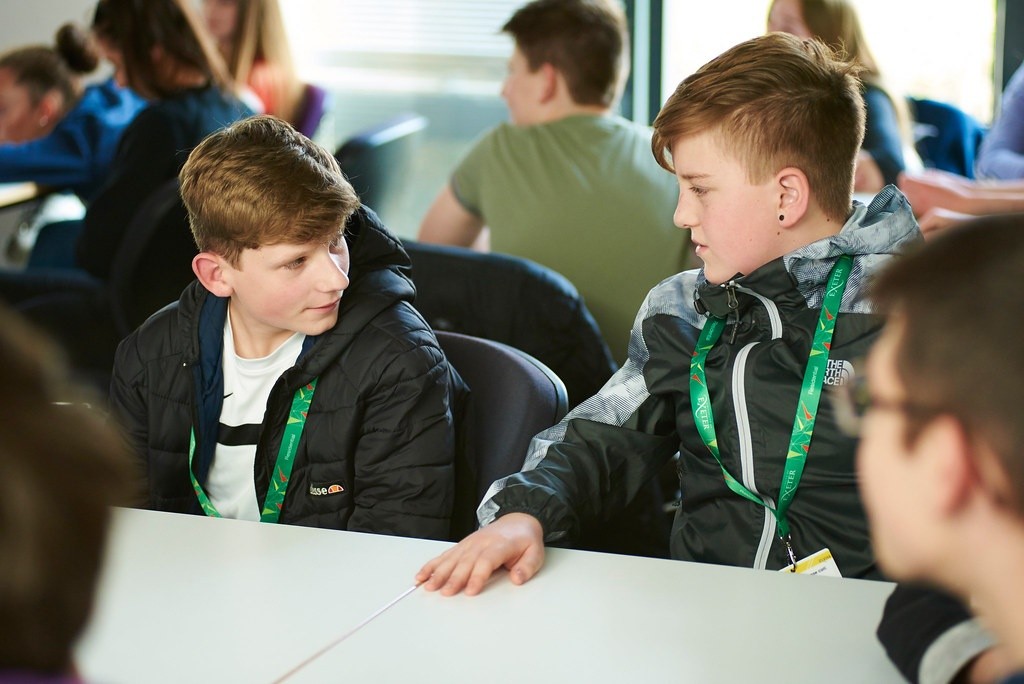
[900,89,989,182]
[401,239,618,541]
[292,77,429,221]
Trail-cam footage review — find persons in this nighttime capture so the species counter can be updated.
[417,32,927,594]
[0,210,1024,684]
[0,0,1024,396]
[110,117,471,549]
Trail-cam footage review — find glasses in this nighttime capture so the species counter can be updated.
[831,378,952,438]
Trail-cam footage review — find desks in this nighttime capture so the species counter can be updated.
[74,504,924,684]
